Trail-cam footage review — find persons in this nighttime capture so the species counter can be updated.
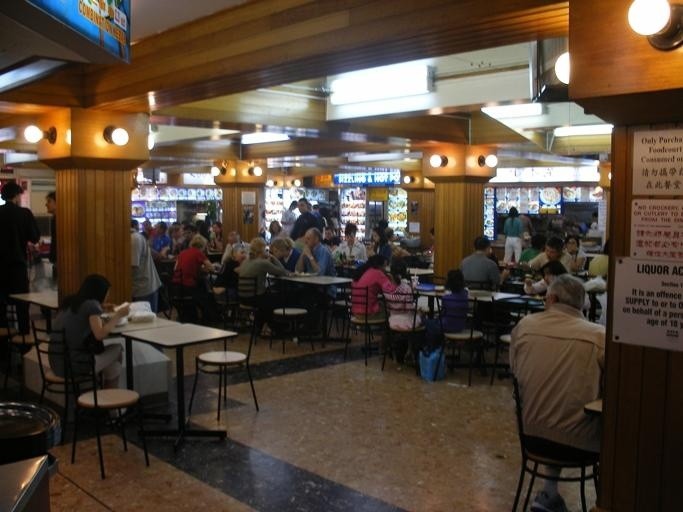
[47,272,129,424]
[509,272,606,512]
[127,197,610,370]
[45,191,57,287]
[1,180,41,343]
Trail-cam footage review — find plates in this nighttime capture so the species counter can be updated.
[295,273,317,276]
[416,282,437,292]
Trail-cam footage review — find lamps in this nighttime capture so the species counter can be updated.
[554,52,570,85]
[430,155,448,168]
[103,126,129,146]
[24,125,57,144]
[291,179,301,187]
[266,180,277,187]
[478,154,498,168]
[211,166,226,176]
[628,0,683,50]
[404,176,414,184]
[249,166,262,176]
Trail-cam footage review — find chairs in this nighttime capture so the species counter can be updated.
[209,270,239,329]
[490,296,529,386]
[234,275,272,345]
[432,295,487,387]
[2,300,38,392]
[30,319,105,444]
[343,284,387,366]
[167,268,201,324]
[380,288,427,377]
[512,377,601,512]
[1,328,17,364]
[267,277,314,354]
[64,346,149,480]
[188,313,259,421]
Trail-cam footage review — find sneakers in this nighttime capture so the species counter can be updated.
[530,491,568,511]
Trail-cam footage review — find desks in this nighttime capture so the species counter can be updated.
[11,291,60,334]
[281,276,354,348]
[122,323,239,454]
[108,317,182,430]
[438,289,521,369]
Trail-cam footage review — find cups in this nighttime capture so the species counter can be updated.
[411,276,418,291]
[435,285,443,293]
